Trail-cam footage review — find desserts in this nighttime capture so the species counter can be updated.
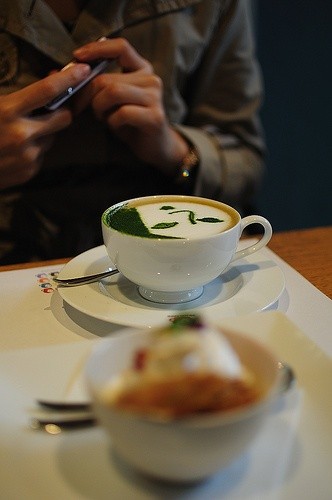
[83,309,286,486]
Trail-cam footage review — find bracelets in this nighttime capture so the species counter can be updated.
[176,152,197,182]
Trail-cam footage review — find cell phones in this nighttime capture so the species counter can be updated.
[44,36,124,113]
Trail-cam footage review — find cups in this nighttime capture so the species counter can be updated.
[101,195,272,303]
[83,322,294,484]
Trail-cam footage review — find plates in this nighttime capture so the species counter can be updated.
[57,244,286,327]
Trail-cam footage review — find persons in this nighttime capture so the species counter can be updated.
[0,0,268,260]
